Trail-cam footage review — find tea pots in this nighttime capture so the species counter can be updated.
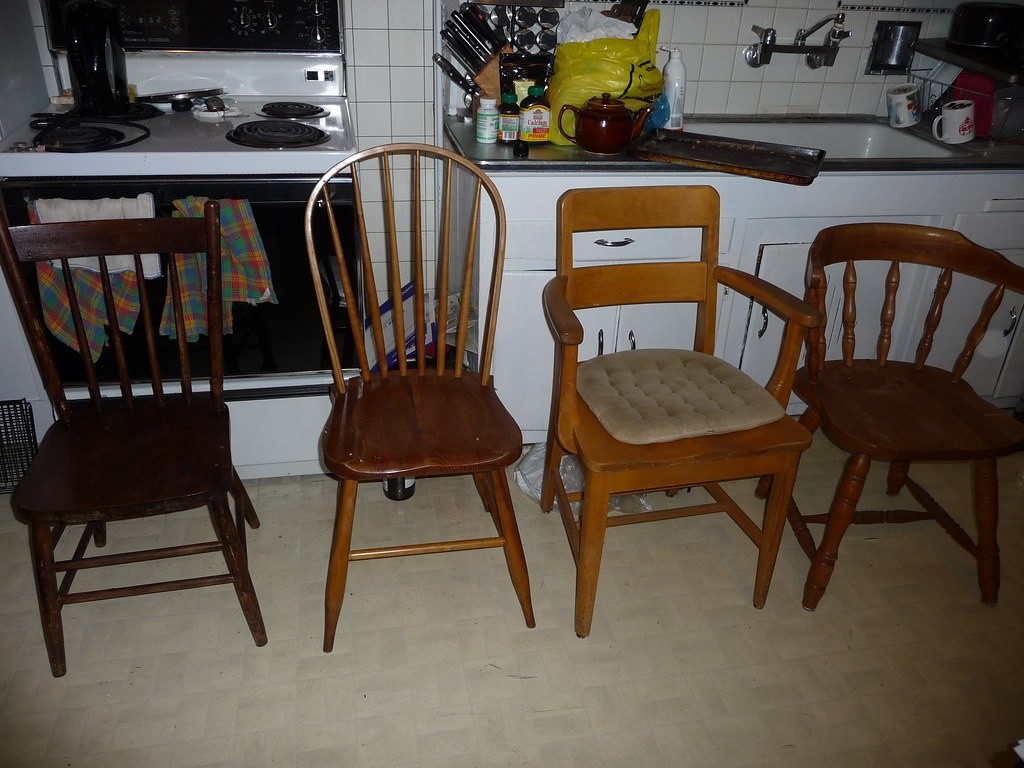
[557,92,655,157]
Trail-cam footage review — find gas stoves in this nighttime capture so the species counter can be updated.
[0,52,357,177]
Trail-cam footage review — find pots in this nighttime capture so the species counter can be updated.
[945,2,1024,71]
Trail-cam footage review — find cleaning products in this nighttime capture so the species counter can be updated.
[658,45,686,131]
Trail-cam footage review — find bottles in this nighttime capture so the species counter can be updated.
[520,87,551,146]
[660,47,686,132]
[382,475,415,502]
[499,93,519,144]
[476,97,499,144]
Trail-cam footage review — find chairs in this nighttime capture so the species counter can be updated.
[307,144,543,650]
[755,223,1024,604]
[1,202,267,676]
[546,186,823,635]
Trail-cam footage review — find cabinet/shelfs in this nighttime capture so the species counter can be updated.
[444,113,1024,445]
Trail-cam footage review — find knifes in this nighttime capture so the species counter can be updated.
[432,2,506,98]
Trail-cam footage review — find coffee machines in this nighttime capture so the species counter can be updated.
[61,1,131,115]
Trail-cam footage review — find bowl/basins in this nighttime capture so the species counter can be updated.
[920,61,963,112]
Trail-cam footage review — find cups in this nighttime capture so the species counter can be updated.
[931,99,976,145]
[885,83,922,128]
[991,84,1024,140]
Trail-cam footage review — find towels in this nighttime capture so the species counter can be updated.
[33,192,162,280]
[172,195,280,307]
[27,200,141,364]
[158,197,234,342]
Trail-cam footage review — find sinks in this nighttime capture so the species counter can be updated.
[683,121,955,158]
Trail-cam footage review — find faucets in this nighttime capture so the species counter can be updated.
[745,12,852,70]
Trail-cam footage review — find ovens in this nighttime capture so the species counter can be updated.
[0,180,363,388]
